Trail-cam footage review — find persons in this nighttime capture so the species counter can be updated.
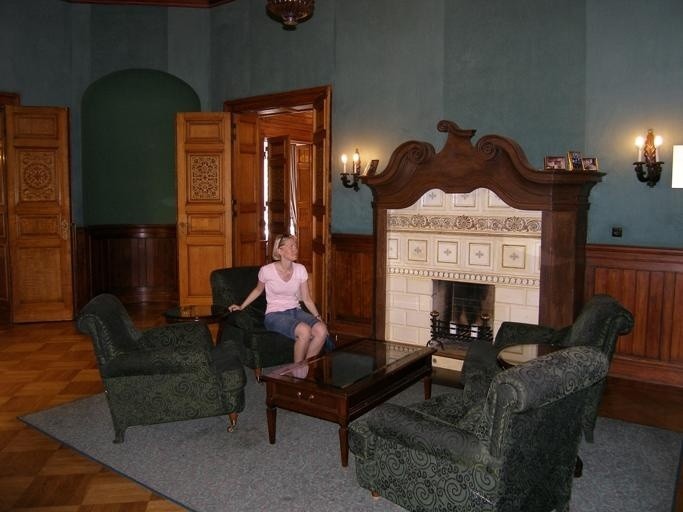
[228,234,330,365]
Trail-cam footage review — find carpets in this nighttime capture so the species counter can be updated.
[15,365,682,512]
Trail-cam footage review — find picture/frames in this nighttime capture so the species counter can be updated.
[567,151,584,171]
[362,160,379,176]
[544,156,565,169]
[582,158,598,171]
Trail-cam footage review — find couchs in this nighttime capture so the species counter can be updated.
[76,294,247,444]
[460,293,635,444]
[210,265,319,383]
[346,345,610,512]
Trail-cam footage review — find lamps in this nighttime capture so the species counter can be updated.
[265,0,314,27]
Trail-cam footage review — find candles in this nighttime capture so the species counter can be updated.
[352,153,360,173]
[341,154,348,174]
[653,136,662,162]
[635,136,646,162]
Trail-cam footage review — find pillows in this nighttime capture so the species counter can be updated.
[497,342,562,370]
